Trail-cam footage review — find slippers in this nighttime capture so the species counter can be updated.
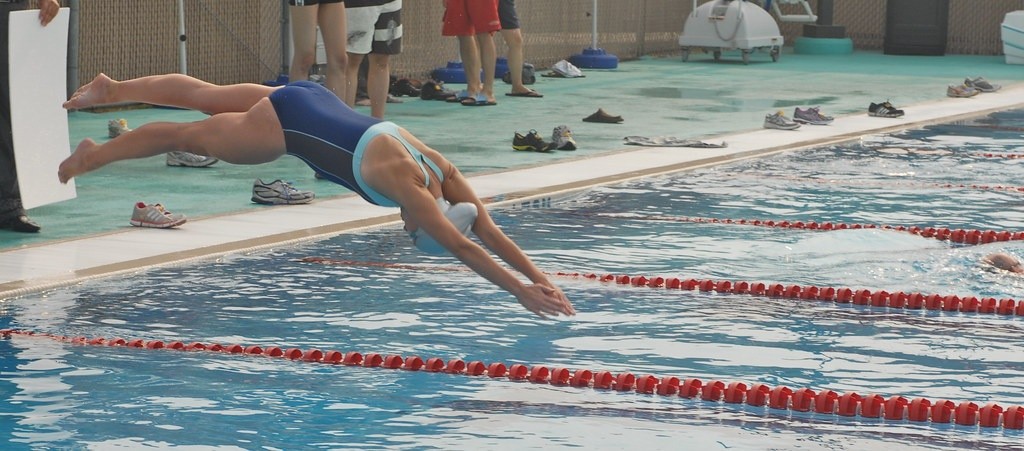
[540,59,587,79]
[582,108,625,123]
[446,89,498,106]
[504,88,545,99]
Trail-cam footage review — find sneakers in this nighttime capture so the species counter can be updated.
[107,118,135,138]
[946,76,1002,98]
[166,151,220,168]
[867,98,906,119]
[512,128,560,153]
[503,63,536,85]
[249,178,317,206]
[129,201,188,229]
[552,125,578,150]
[792,106,836,125]
[762,110,802,130]
[387,73,455,100]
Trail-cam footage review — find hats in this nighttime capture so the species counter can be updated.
[414,202,481,254]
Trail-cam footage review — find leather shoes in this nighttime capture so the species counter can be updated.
[2,213,43,233]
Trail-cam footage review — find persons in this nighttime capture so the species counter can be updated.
[290,0,404,121]
[0,0,59,232]
[58,73,576,317]
[983,252,1024,273]
[441,0,544,106]
